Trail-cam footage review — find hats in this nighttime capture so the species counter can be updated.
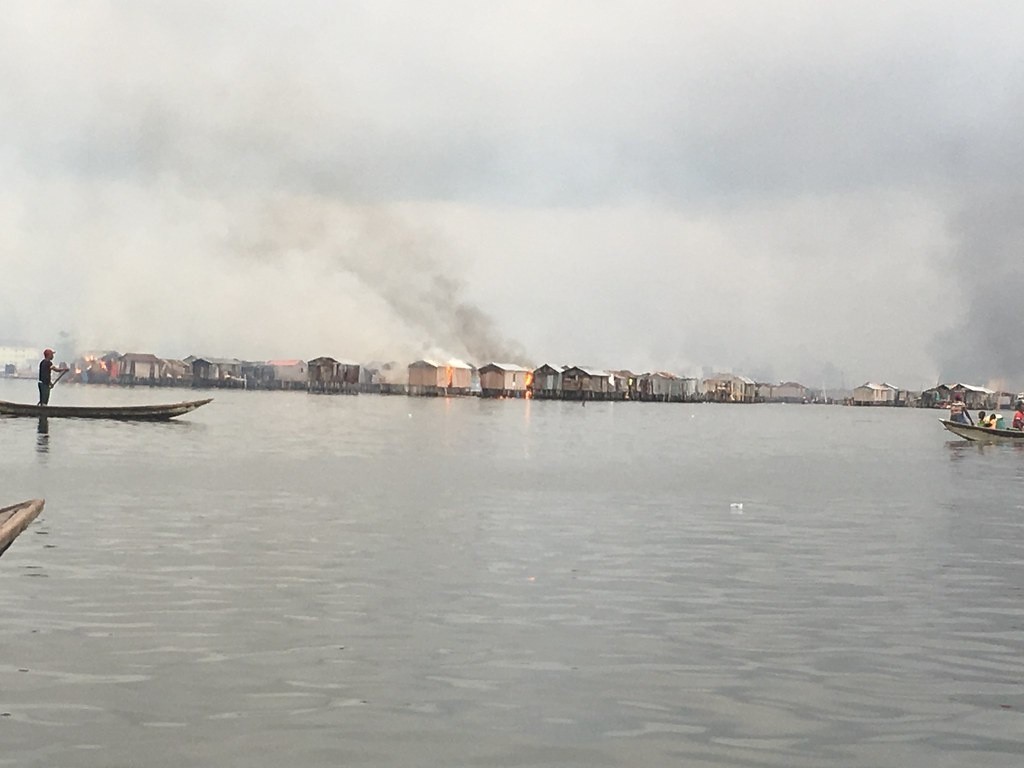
[955,394,961,399]
[44,349,56,357]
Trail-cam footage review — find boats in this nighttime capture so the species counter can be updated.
[0,499,45,558]
[0,398,214,418]
[937,417,1024,441]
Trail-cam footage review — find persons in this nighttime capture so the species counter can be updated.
[1012,403,1024,431]
[950,395,975,426]
[37,348,70,407]
[977,411,996,428]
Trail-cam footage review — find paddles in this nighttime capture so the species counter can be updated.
[35,369,70,406]
